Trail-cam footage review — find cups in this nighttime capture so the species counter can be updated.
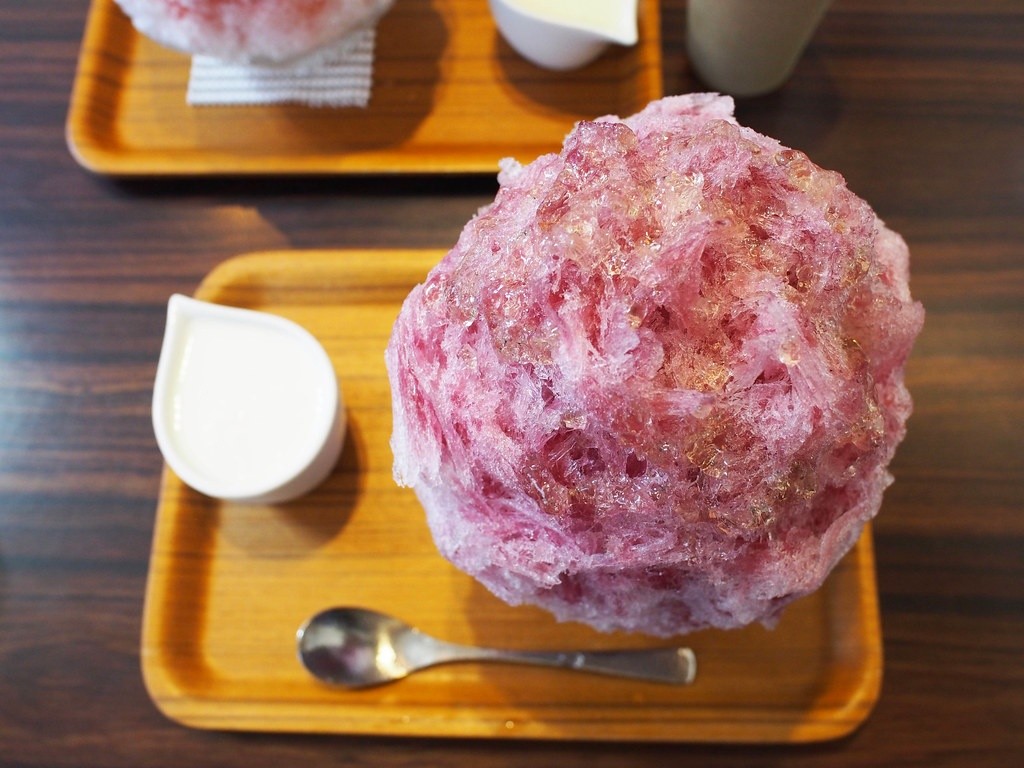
[493,0,640,74]
[685,1,828,99]
[151,292,346,504]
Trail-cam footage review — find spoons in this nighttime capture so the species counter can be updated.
[295,606,696,688]
